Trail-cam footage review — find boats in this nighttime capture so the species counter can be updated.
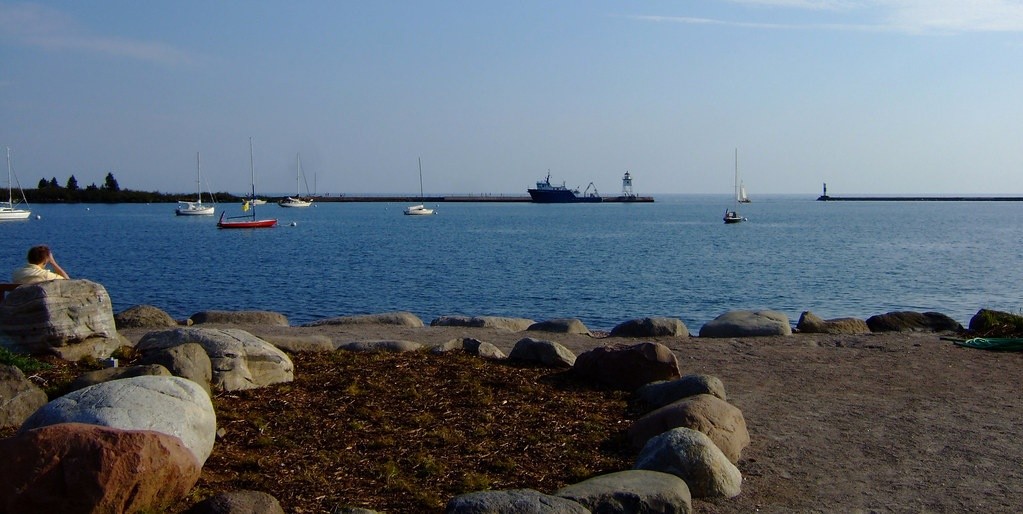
[241,192,269,205]
[734,146,752,203]
[527,167,581,203]
[723,208,748,224]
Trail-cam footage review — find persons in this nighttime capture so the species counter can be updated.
[11,245,70,283]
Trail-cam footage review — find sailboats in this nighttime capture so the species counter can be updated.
[276,152,314,208]
[402,155,433,215]
[0,145,32,219]
[174,152,215,216]
[215,137,279,229]
